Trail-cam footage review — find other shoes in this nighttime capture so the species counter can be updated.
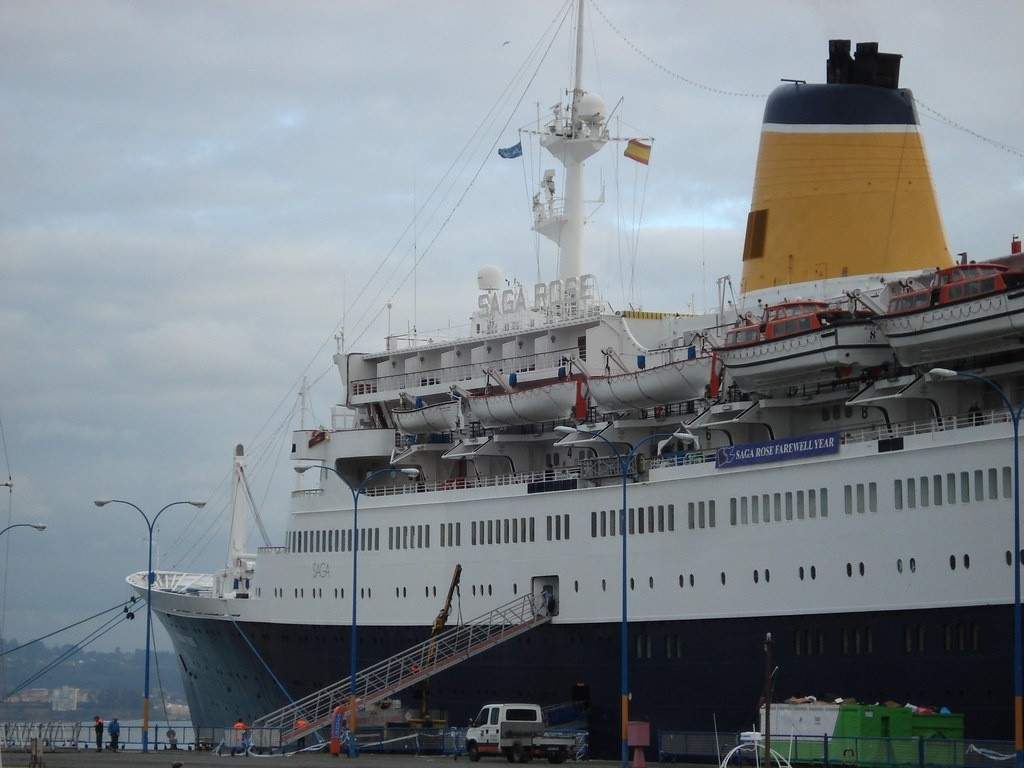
[96,750,101,752]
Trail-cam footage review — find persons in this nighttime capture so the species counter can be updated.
[234,719,251,746]
[94,716,104,752]
[537,590,556,616]
[295,716,308,753]
[107,717,120,751]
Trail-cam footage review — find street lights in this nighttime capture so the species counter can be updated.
[93,498,208,755]
[552,426,697,768]
[928,368,1024,768]
[292,464,424,759]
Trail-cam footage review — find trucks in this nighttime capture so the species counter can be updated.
[464,702,575,765]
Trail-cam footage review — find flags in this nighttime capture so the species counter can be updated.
[498,142,522,158]
[623,140,651,164]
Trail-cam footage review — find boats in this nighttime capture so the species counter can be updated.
[586,348,725,413]
[389,390,466,436]
[463,365,588,429]
[711,297,896,385]
[848,262,1024,370]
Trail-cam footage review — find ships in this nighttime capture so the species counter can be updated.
[126,17,1024,747]
